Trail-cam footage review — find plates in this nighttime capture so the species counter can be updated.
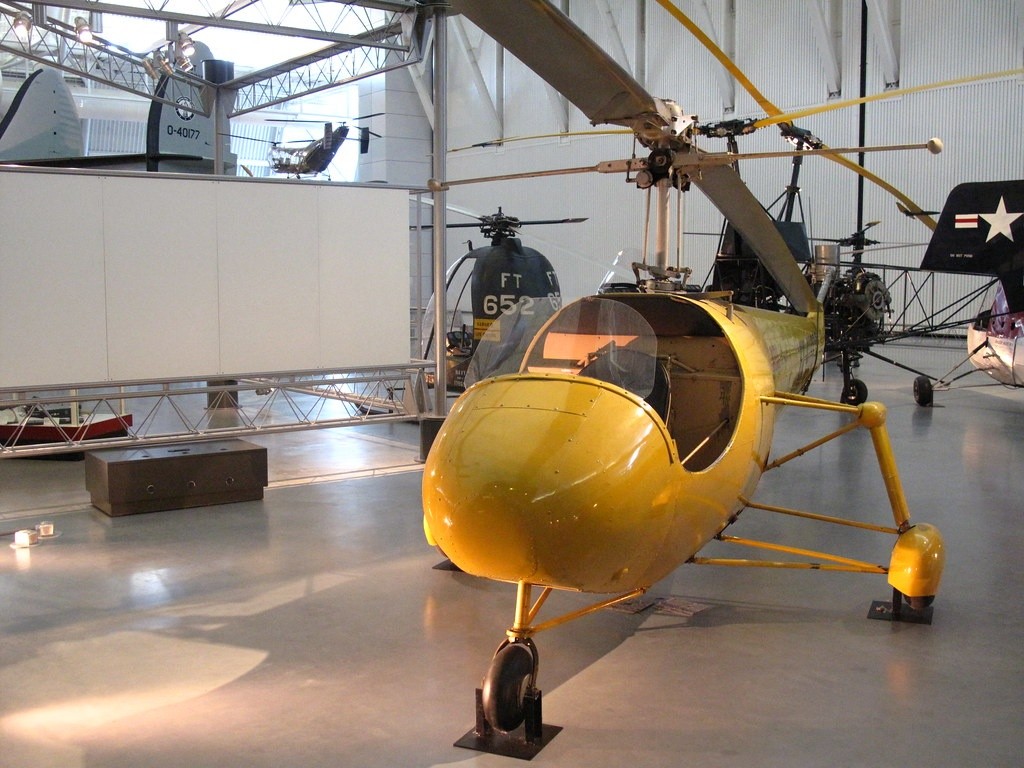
[9,541,43,549]
[36,530,63,539]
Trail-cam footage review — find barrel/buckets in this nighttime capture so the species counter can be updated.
[814,244,841,282]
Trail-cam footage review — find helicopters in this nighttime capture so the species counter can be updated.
[263,111,386,180]
[407,0,1024,768]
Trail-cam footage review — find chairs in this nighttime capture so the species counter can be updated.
[578,350,672,428]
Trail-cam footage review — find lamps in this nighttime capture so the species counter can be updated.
[14,16,32,34]
[141,33,196,79]
[73,16,92,42]
